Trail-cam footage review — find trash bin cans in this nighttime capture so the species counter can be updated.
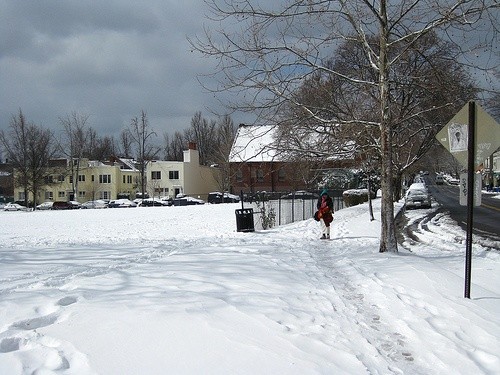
[235,208,255,232]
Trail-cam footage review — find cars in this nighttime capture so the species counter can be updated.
[421,170,458,185]
[288,190,319,199]
[36,192,241,211]
[3,204,27,211]
[405,189,431,210]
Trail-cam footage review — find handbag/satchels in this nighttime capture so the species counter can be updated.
[326,214,333,223]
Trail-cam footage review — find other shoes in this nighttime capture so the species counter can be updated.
[327,235,330,239]
[320,236,326,239]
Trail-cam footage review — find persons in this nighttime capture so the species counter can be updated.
[318,188,335,239]
